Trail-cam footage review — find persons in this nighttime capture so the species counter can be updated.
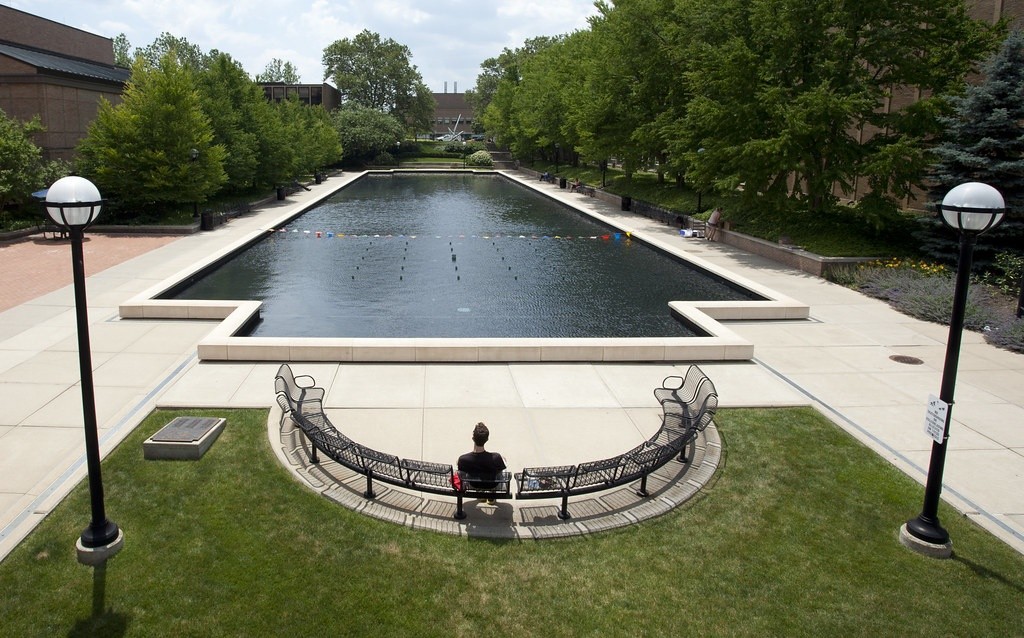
[569,178,580,193]
[707,206,723,242]
[458,424,507,505]
[539,170,548,181]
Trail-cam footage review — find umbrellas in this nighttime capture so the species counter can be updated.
[31,189,48,198]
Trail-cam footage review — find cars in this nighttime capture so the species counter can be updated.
[476,135,485,141]
[436,134,451,142]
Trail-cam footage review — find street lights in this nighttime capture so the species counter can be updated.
[396,142,401,168]
[462,141,466,169]
[192,148,201,217]
[32,175,128,568]
[696,148,707,213]
[555,143,560,173]
[894,182,1010,563]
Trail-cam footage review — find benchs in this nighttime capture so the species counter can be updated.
[575,183,594,197]
[540,173,555,183]
[274,363,718,522]
[631,200,685,229]
